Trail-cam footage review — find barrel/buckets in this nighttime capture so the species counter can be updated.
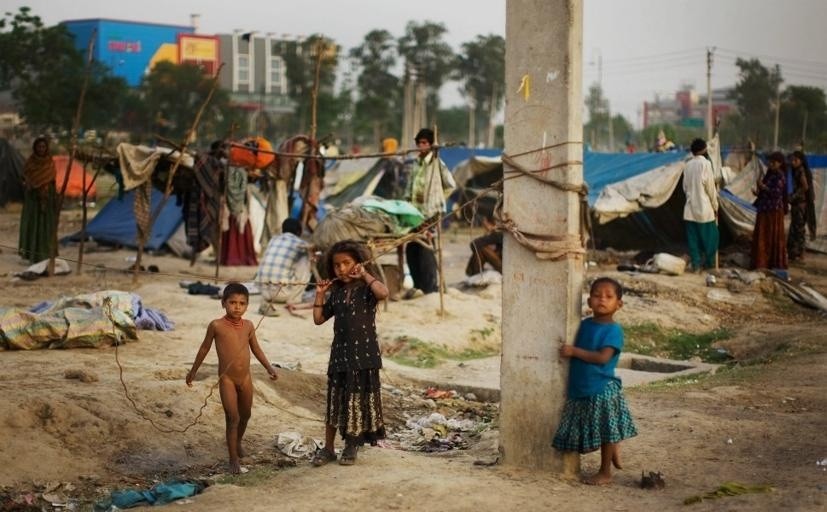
[651,251,687,276]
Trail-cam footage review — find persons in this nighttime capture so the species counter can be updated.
[681,137,724,279]
[787,150,817,265]
[312,236,389,467]
[183,142,260,266]
[288,137,325,226]
[16,137,60,264]
[749,149,790,271]
[319,135,399,155]
[550,276,638,485]
[465,214,502,276]
[184,282,276,474]
[257,218,316,306]
[98,120,502,314]
[403,126,447,235]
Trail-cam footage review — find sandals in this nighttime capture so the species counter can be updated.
[312,447,338,466]
[340,446,358,466]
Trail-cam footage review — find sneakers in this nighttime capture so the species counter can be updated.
[257,301,281,317]
[178,278,219,294]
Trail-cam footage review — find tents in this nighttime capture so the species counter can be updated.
[63,186,276,264]
[319,155,408,211]
[438,146,502,218]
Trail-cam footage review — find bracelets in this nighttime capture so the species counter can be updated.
[367,279,377,289]
[313,303,324,307]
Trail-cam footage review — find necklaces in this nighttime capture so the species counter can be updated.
[222,315,244,329]
